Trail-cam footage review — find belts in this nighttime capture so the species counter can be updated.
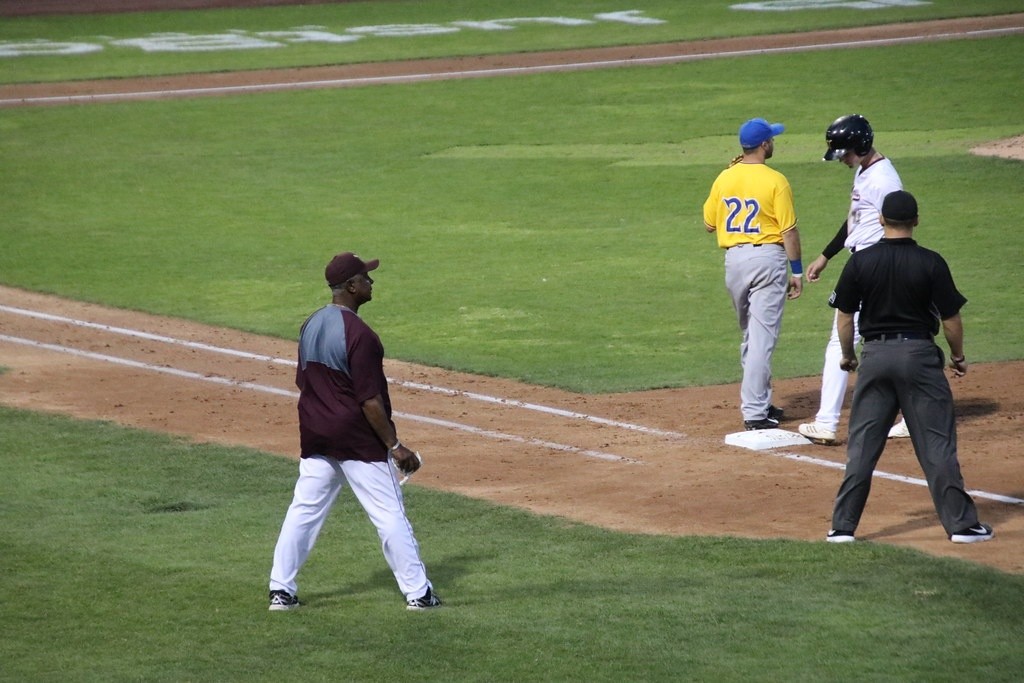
[850,245,856,253]
[863,331,935,342]
[726,242,785,250]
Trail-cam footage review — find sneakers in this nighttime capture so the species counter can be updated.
[769,405,783,418]
[888,418,910,438]
[406,587,443,611]
[269,590,301,611]
[826,529,855,544]
[744,418,779,431]
[798,423,836,440]
[951,522,994,543]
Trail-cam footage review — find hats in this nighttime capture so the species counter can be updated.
[882,190,918,221]
[740,118,785,148]
[325,252,379,288]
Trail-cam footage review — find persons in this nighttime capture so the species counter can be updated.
[702,115,808,432]
[825,191,995,544]
[798,115,906,440]
[269,251,444,612]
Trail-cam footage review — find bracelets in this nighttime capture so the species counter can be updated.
[949,354,965,364]
[388,440,401,452]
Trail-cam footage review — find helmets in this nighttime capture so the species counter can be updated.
[823,114,874,161]
